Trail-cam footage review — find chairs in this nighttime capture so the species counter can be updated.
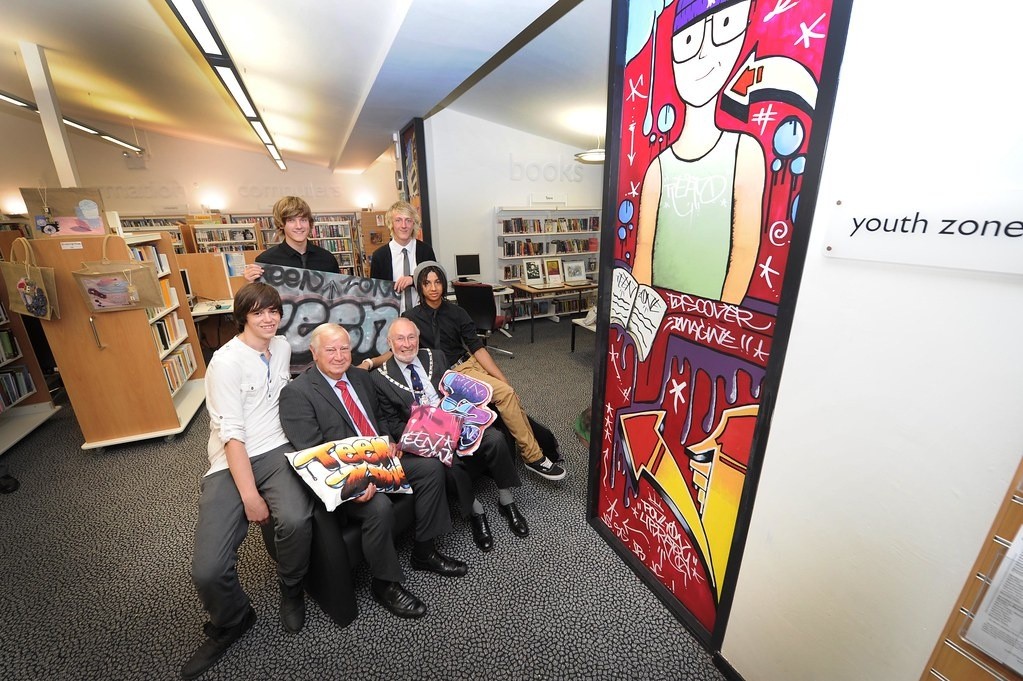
[450,280,514,359]
[570,318,597,351]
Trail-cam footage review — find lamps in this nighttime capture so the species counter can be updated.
[574,135,605,162]
[0,90,145,153]
[166,0,287,171]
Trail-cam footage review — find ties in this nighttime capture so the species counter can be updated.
[334,380,377,439]
[406,364,428,407]
[401,247,413,311]
[432,307,441,352]
[299,253,308,270]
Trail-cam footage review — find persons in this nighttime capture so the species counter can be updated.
[356,261,567,480]
[242,196,340,364]
[371,201,436,316]
[179,281,311,681]
[280,322,467,619]
[370,316,528,553]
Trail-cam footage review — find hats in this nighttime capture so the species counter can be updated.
[413,260,448,290]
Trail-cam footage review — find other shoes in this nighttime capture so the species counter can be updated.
[0,473,20,494]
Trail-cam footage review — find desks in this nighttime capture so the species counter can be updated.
[191,300,235,350]
[510,280,598,343]
[448,288,514,339]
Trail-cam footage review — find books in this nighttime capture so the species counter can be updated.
[194,230,258,253]
[0,223,33,238]
[237,216,361,276]
[498,216,599,319]
[131,245,197,395]
[120,218,186,253]
[0,302,35,413]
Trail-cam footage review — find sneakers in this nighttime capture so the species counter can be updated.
[523,455,566,481]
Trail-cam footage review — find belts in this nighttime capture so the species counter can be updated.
[450,352,470,371]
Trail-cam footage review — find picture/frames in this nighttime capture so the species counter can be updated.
[399,118,431,245]
[523,258,592,290]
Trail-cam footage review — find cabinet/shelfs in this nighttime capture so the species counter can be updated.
[495,203,603,330]
[0,212,391,456]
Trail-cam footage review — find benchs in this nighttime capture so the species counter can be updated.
[261,405,516,626]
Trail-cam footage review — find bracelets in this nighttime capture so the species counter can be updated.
[362,358,373,370]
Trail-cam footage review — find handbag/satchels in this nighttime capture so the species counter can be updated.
[71,234,166,315]
[0,237,62,322]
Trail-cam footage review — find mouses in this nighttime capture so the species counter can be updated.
[215,305,221,309]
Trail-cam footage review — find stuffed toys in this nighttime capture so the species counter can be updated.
[17,277,47,317]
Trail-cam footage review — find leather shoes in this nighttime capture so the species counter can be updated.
[470,513,493,552]
[180,606,259,680]
[497,496,530,537]
[409,546,470,579]
[370,578,427,618]
[277,578,305,634]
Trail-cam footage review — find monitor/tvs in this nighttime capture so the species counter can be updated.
[454,253,481,282]
[179,269,191,297]
